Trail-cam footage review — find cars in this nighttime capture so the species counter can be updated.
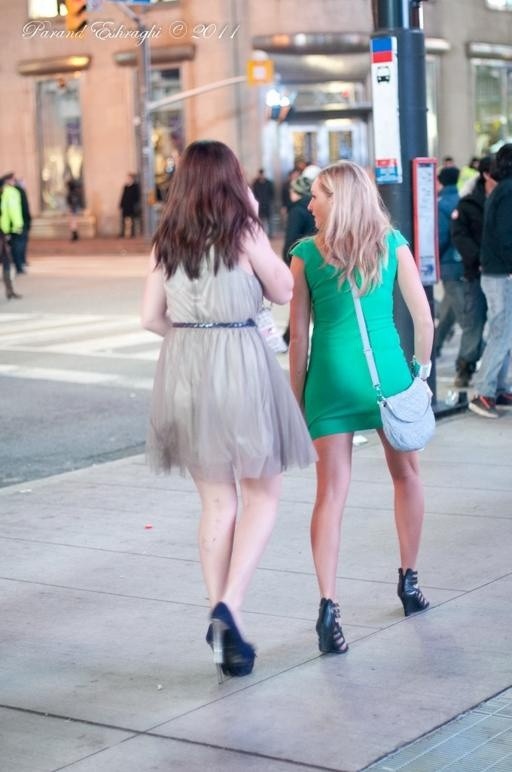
[376,65,392,84]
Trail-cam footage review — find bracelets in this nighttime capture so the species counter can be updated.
[411,355,432,379]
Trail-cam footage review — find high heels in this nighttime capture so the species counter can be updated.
[206,601,255,685]
[397,568,429,617]
[316,598,349,654]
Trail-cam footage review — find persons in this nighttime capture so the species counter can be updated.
[116,172,140,239]
[436,142,511,419]
[253,159,320,344]
[141,141,295,686]
[1,171,30,300]
[290,160,434,651]
[67,175,84,241]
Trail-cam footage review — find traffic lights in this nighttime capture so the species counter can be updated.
[247,59,273,86]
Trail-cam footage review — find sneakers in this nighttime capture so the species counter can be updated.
[469,394,499,419]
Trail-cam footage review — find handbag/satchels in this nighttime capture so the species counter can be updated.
[377,376,437,452]
